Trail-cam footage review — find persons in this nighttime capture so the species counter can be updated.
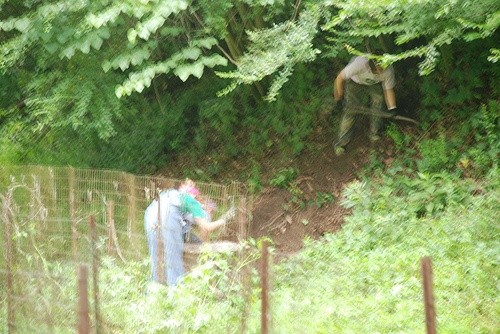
[334,48,397,149]
[177,180,235,256]
[144,188,205,288]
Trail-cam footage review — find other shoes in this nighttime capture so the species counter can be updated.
[371,135,380,142]
[335,144,345,156]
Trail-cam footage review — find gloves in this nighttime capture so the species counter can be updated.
[388,106,399,122]
[336,100,343,111]
